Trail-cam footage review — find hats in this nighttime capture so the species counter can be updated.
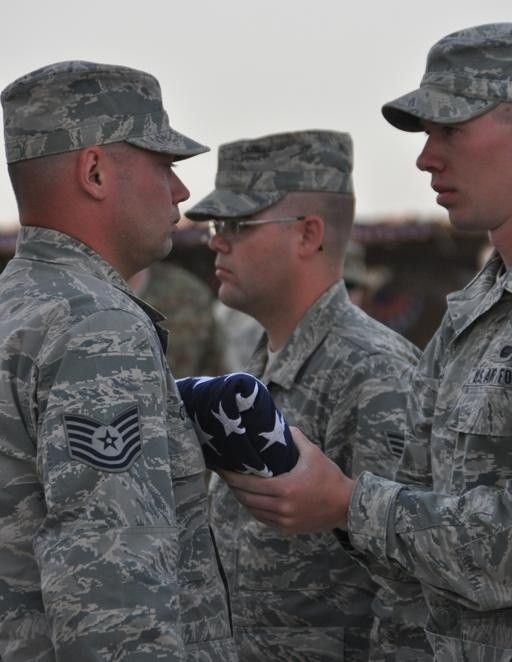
[0,60,211,165]
[184,130,355,223]
[381,22,512,133]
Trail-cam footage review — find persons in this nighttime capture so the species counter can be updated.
[184,130,423,662]
[0,63,237,662]
[220,23,512,662]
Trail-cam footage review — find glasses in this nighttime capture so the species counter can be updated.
[208,216,324,251]
